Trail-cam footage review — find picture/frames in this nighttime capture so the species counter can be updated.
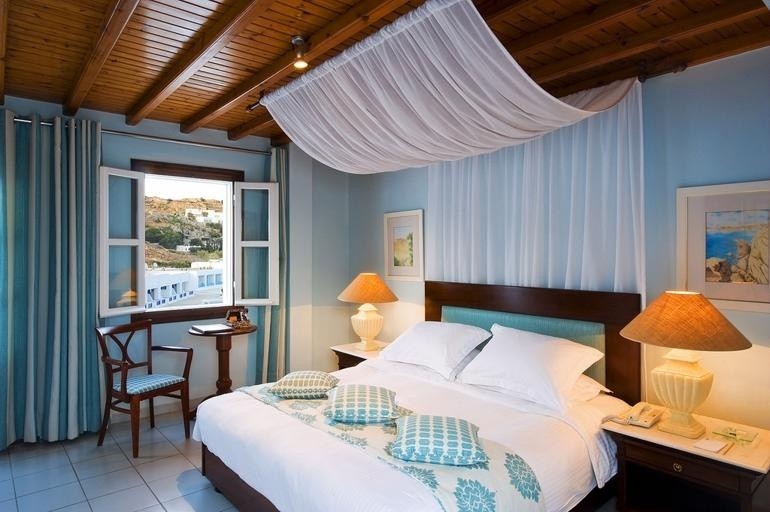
[382,209,425,282]
[673,178,770,314]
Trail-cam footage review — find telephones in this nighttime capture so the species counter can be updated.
[626,401,667,429]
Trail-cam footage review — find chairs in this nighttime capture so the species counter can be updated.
[95,319,194,459]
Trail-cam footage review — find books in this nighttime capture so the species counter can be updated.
[693,437,727,454]
[190,324,238,335]
[718,440,734,456]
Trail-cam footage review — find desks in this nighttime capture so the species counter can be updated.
[186,323,257,418]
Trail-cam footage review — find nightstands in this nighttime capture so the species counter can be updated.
[331,339,391,370]
[601,402,770,512]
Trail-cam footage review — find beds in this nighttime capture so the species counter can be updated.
[187,281,641,512]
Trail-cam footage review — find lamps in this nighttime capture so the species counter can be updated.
[620,289,752,438]
[291,34,310,69]
[337,272,399,350]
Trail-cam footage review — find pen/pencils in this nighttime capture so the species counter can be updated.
[722,442,736,456]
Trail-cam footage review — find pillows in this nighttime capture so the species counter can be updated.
[368,348,481,385]
[382,321,492,379]
[470,372,613,411]
[268,370,340,400]
[391,414,488,466]
[455,323,605,413]
[323,384,396,423]
